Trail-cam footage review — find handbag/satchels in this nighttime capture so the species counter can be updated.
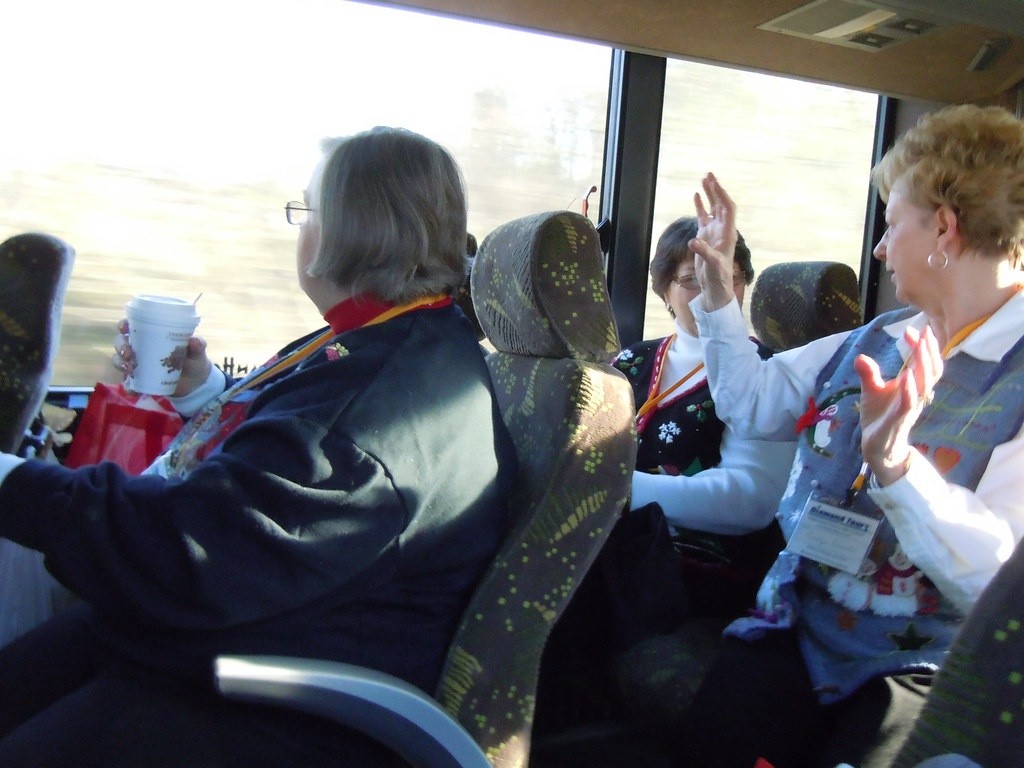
[65,382,184,477]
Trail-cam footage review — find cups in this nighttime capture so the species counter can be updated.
[123,295,201,396]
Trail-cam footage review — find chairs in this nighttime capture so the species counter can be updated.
[0,233,75,456]
[102,208,638,768]
[750,262,862,352]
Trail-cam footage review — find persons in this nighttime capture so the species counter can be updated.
[534,105,1023,768]
[1,128,524,768]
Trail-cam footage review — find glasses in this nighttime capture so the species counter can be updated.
[671,269,747,290]
[284,201,319,225]
